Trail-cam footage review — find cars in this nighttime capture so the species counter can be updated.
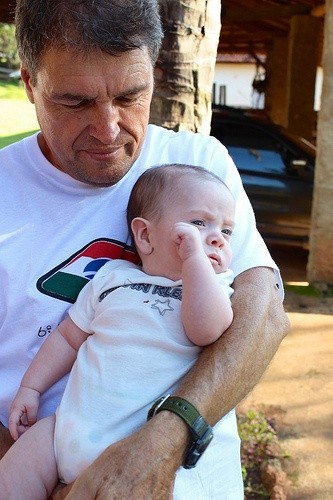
[212,104,316,250]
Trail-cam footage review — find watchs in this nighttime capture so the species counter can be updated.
[146,395,214,470]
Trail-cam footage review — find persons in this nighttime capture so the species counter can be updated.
[1,162,236,499]
[1,0,290,500]
